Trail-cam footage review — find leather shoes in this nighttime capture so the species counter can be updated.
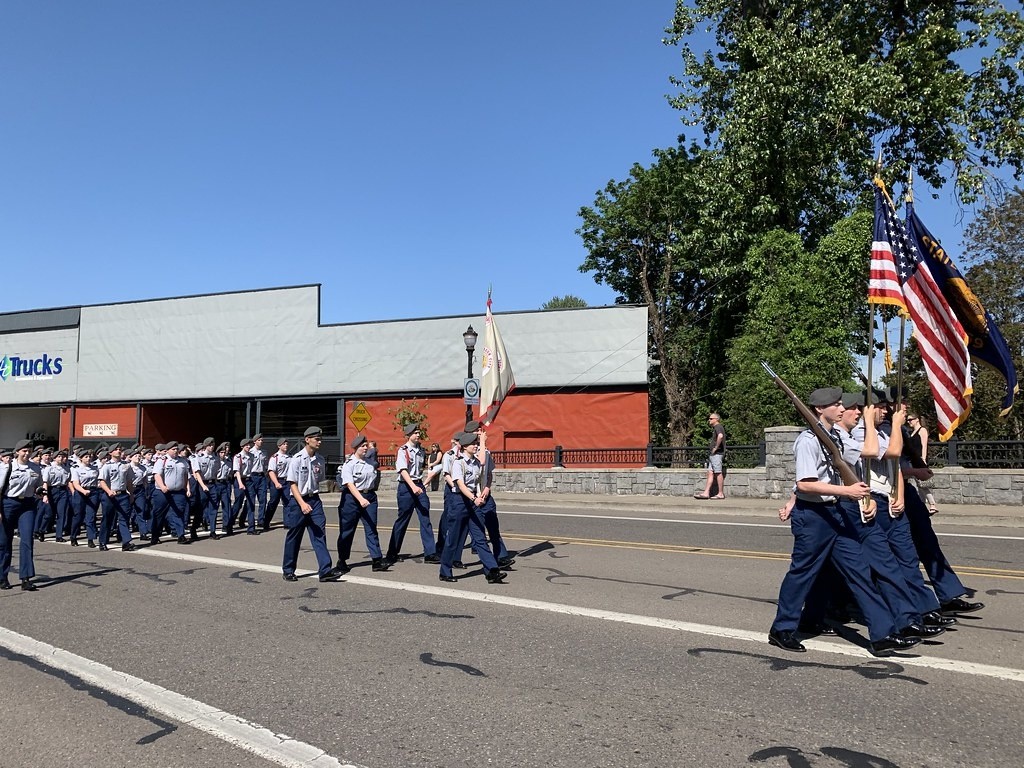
[870,632,923,656]
[0,580,13,589]
[386,555,404,561]
[439,549,516,584]
[22,581,36,590]
[13,522,271,551]
[921,611,959,627]
[939,598,985,616]
[899,623,947,639]
[282,572,298,581]
[319,569,341,582]
[372,561,392,572]
[424,552,441,564]
[798,611,842,636]
[826,606,858,623]
[768,627,807,652]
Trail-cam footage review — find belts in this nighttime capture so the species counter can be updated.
[826,500,841,505]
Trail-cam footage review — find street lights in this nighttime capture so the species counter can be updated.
[462,325,477,421]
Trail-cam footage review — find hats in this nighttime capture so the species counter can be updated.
[808,387,842,406]
[0,433,290,459]
[304,423,420,449]
[453,421,479,447]
[842,386,909,409]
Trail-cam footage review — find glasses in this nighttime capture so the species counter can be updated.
[709,417,718,420]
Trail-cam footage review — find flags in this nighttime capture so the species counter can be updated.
[477,300,516,430]
[867,177,1020,444]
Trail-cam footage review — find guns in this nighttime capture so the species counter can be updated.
[760,359,860,486]
[845,358,928,468]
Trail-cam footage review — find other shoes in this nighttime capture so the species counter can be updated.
[337,560,349,572]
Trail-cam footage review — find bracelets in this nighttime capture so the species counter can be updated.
[470,495,477,502]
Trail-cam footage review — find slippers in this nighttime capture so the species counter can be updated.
[694,493,710,499]
[710,493,725,499]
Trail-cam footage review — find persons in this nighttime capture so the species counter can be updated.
[0,439,44,591]
[0,432,295,552]
[435,420,515,584]
[364,441,380,491]
[282,426,344,582]
[768,385,986,658]
[337,435,392,572]
[693,413,727,500]
[386,422,442,564]
[423,442,444,490]
